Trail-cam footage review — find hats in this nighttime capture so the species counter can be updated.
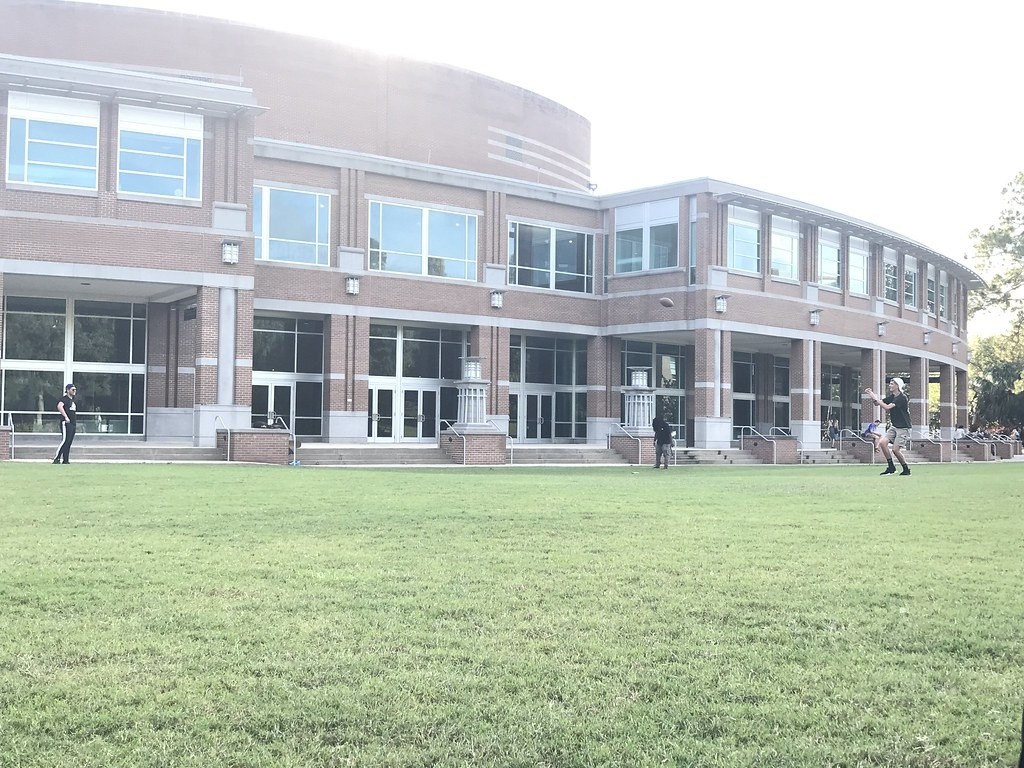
[892,378,904,393]
[65,384,75,393]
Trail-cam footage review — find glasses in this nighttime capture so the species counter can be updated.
[70,389,77,391]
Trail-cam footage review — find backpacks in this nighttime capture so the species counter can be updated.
[835,428,838,434]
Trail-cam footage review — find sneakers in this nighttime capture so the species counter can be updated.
[881,467,898,476]
[900,470,912,476]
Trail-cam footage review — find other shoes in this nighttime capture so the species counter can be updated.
[62,460,70,464]
[831,446,835,448]
[52,459,60,464]
[875,448,880,453]
[663,467,669,470]
[654,466,660,468]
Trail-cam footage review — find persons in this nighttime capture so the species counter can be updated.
[829,417,1024,453]
[52,384,77,464]
[864,377,912,475]
[653,416,674,469]
